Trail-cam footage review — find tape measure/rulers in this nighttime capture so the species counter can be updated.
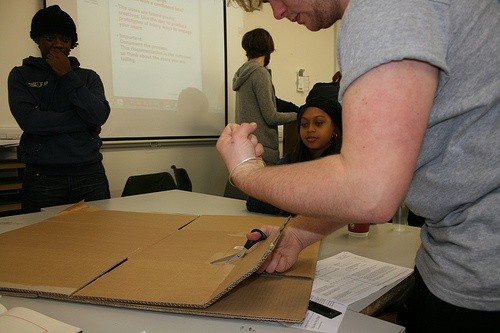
[307,300,342,319]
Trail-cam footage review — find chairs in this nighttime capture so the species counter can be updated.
[171,165,193,192]
[122,171,177,196]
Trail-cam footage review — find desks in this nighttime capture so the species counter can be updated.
[0,189,422,333]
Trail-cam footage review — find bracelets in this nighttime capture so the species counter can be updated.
[227,156,266,187]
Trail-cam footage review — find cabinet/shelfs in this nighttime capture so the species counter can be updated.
[0,144,26,217]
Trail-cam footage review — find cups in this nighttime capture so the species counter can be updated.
[347,223,370,237]
[392,203,409,232]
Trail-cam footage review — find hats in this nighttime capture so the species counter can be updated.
[30,4,77,43]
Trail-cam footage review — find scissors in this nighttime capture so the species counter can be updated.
[211,229,267,265]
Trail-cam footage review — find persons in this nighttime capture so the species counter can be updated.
[246,100,342,215]
[7,5,111,214]
[222,28,298,199]
[216,0,500,333]
